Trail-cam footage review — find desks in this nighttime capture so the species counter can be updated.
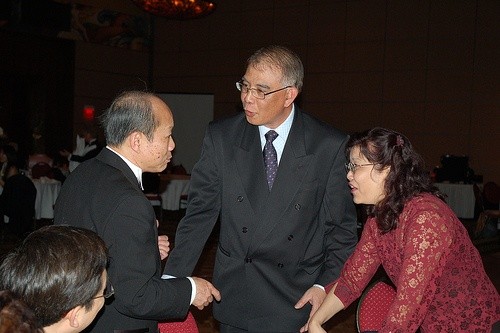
[0,168,62,224]
[144,174,192,211]
[434,182,484,224]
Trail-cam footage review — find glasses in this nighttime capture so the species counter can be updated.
[92,279,115,299]
[235,79,293,100]
[343,162,383,172]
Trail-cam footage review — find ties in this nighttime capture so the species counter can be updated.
[263,130,279,193]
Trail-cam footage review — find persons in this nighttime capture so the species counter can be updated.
[160,45,361,333]
[308,127,500,333]
[0,226,108,333]
[0,125,100,239]
[0,289,45,333]
[55,91,221,333]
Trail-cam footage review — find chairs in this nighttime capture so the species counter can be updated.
[355,281,397,333]
[0,150,69,249]
[149,304,202,333]
[142,165,188,225]
[474,182,500,239]
